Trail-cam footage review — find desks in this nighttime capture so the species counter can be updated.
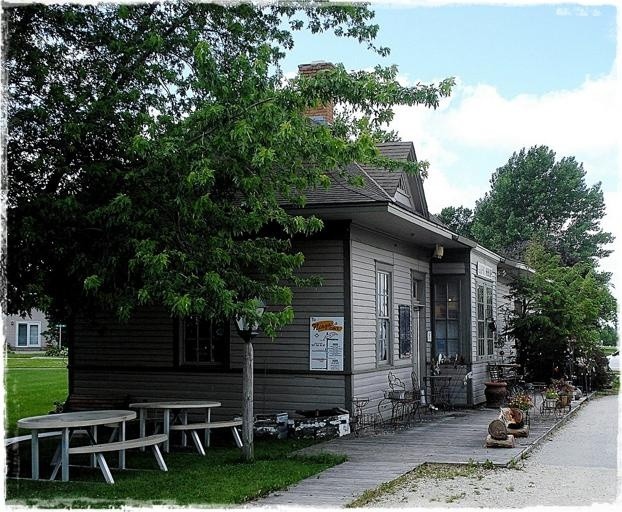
[16,399,222,482]
[379,397,420,431]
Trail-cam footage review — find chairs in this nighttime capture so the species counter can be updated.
[387,370,436,423]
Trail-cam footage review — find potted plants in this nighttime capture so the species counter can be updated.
[544,386,559,408]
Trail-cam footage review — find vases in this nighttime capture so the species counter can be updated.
[484,381,508,408]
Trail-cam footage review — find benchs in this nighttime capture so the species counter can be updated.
[5,417,242,484]
